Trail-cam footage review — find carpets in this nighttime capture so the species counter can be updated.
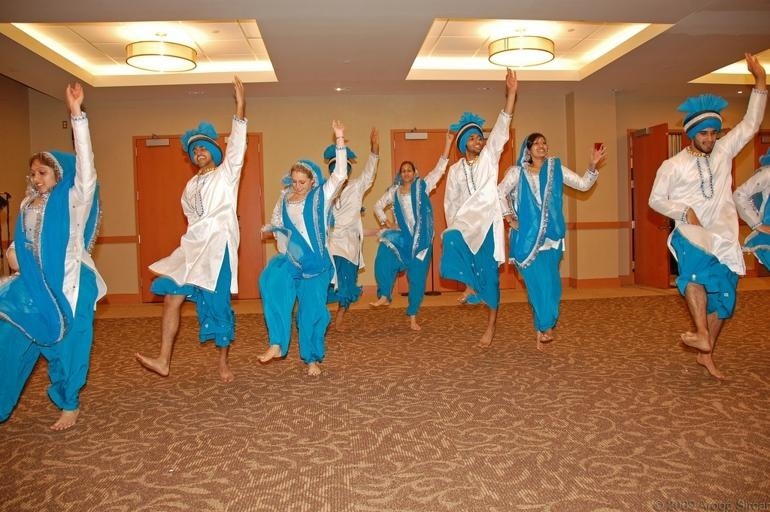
[0,289,770,510]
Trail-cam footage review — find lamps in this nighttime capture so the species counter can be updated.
[488,35,555,68]
[126,40,197,74]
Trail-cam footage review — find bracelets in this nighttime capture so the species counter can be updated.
[336,137,346,141]
[508,218,514,224]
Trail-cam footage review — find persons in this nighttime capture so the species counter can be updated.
[442,70,517,349]
[327,130,379,333]
[0,80,101,432]
[374,130,454,330]
[135,75,245,384]
[497,133,609,351]
[648,53,767,379]
[255,120,347,375]
[732,148,770,271]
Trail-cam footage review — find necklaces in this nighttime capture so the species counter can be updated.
[193,166,217,216]
[463,155,479,195]
[333,196,341,211]
[687,148,714,199]
